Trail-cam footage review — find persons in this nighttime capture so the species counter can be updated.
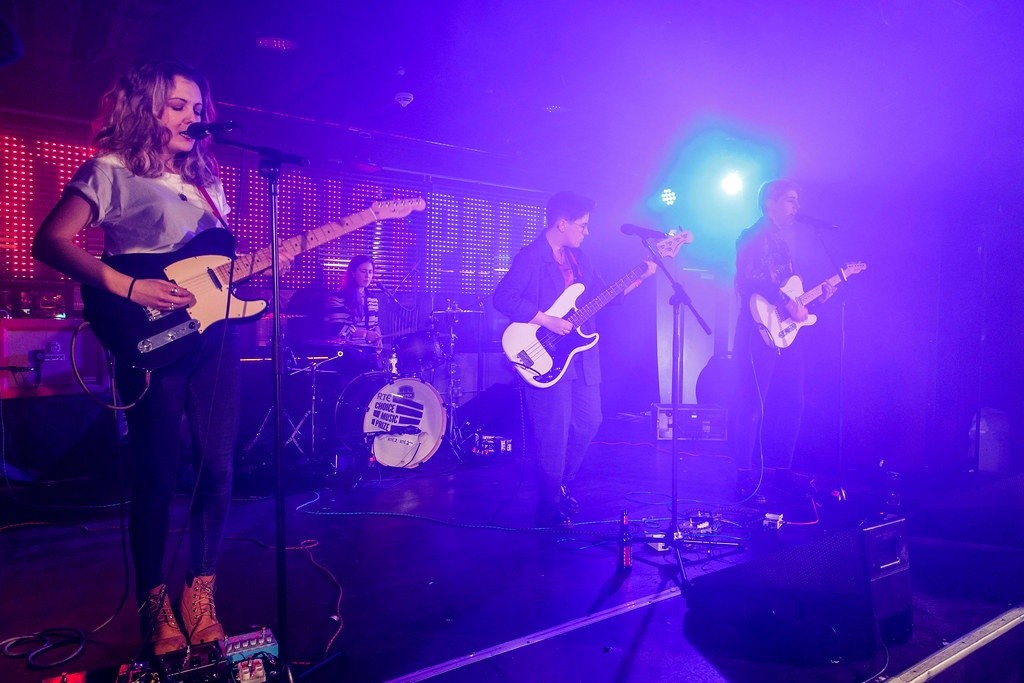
[731,178,838,506]
[31,53,295,656]
[324,255,383,347]
[493,191,657,529]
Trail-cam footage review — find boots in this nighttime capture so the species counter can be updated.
[174,573,224,644]
[140,583,186,655]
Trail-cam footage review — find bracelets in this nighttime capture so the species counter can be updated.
[635,273,647,287]
[128,278,136,301]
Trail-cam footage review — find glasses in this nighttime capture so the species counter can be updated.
[563,217,588,232]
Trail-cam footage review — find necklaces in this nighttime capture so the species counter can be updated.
[166,175,188,202]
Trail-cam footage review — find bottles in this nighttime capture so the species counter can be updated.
[389,348,398,379]
[616,510,632,573]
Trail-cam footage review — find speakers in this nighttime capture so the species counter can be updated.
[1,317,120,491]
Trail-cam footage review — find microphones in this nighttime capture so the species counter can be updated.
[31,349,45,385]
[620,224,668,240]
[794,212,838,229]
[187,121,239,140]
[377,282,398,303]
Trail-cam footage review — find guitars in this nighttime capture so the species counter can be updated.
[748,261,867,349]
[81,194,428,378]
[499,225,693,390]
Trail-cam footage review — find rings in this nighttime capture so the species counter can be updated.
[284,265,291,270]
[171,287,178,296]
[563,329,567,333]
[169,303,175,311]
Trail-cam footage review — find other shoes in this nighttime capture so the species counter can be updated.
[561,484,579,508]
[536,507,570,523]
[773,477,802,497]
[734,484,766,503]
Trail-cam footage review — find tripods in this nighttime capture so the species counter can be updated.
[443,314,493,464]
[606,239,744,589]
[244,345,347,456]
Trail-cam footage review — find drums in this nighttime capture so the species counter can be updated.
[391,328,446,374]
[335,370,448,470]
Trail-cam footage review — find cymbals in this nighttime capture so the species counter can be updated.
[433,309,486,315]
[259,313,305,320]
[302,338,378,349]
